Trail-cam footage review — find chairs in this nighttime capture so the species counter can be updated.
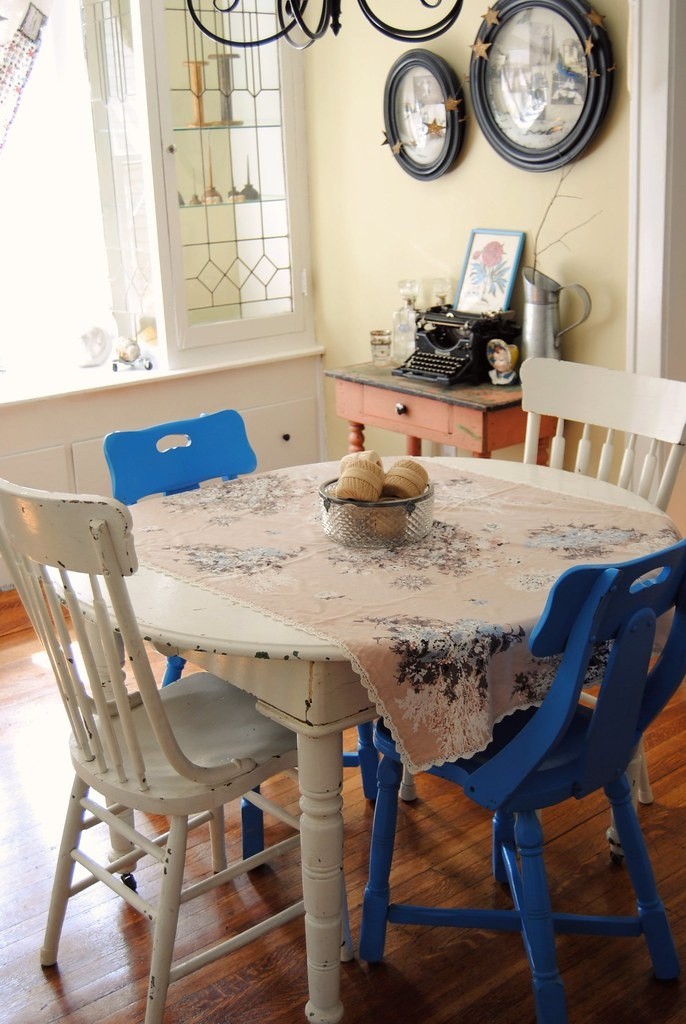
[359,540,686,1024]
[0,480,352,1024]
[397,355,686,856]
[104,410,381,868]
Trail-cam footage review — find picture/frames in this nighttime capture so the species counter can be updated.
[468,0,614,173]
[383,48,467,182]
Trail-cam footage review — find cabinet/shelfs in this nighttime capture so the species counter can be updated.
[324,358,567,466]
[173,123,286,208]
[0,354,320,589]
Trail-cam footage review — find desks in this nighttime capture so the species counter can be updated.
[43,455,678,1024]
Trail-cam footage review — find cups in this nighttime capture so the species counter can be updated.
[370,330,392,367]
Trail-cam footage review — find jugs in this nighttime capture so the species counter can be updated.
[522,265,592,361]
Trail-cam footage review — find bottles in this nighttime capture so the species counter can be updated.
[389,280,422,369]
